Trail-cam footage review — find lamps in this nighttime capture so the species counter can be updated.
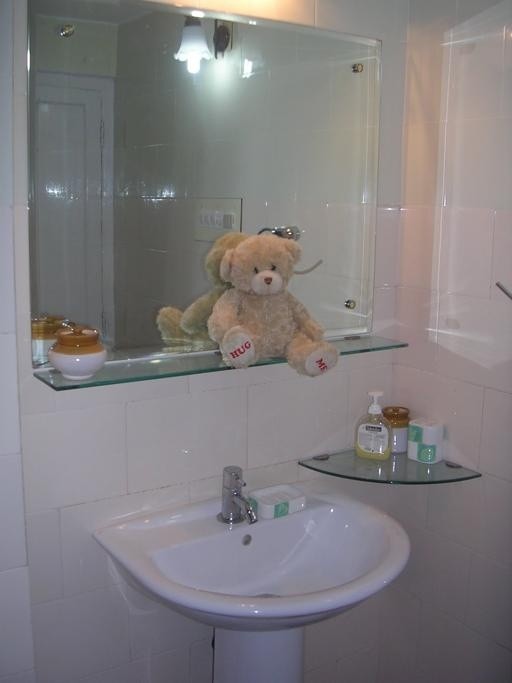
[174,17,213,73]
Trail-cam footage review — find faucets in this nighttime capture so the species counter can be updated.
[215,465,258,525]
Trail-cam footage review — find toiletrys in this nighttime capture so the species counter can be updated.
[354,390,393,462]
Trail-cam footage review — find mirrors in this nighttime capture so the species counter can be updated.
[27,0,409,369]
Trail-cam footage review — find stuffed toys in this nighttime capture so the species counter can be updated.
[207,233,339,379]
[157,233,249,350]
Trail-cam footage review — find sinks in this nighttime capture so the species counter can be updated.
[90,476,412,632]
